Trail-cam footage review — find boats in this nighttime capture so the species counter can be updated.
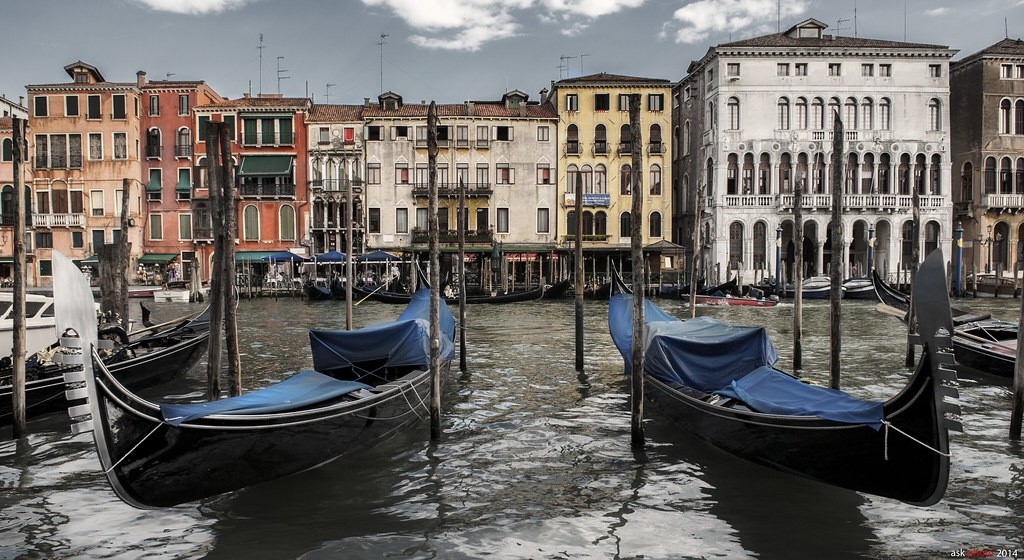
[89,289,457,508]
[609,248,955,506]
[0,291,67,351]
[870,265,1017,379]
[298,267,873,301]
[0,306,228,422]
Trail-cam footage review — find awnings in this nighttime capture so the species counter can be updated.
[138,254,177,264]
[80,255,99,266]
[236,252,279,263]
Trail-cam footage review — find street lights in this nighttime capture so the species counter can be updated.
[979,224,1002,281]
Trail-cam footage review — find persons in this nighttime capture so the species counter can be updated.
[769,275,776,289]
[391,262,400,293]
[746,284,764,299]
[851,265,857,277]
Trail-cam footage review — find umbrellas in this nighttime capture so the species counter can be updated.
[262,251,302,261]
[312,251,346,262]
[358,251,402,261]
